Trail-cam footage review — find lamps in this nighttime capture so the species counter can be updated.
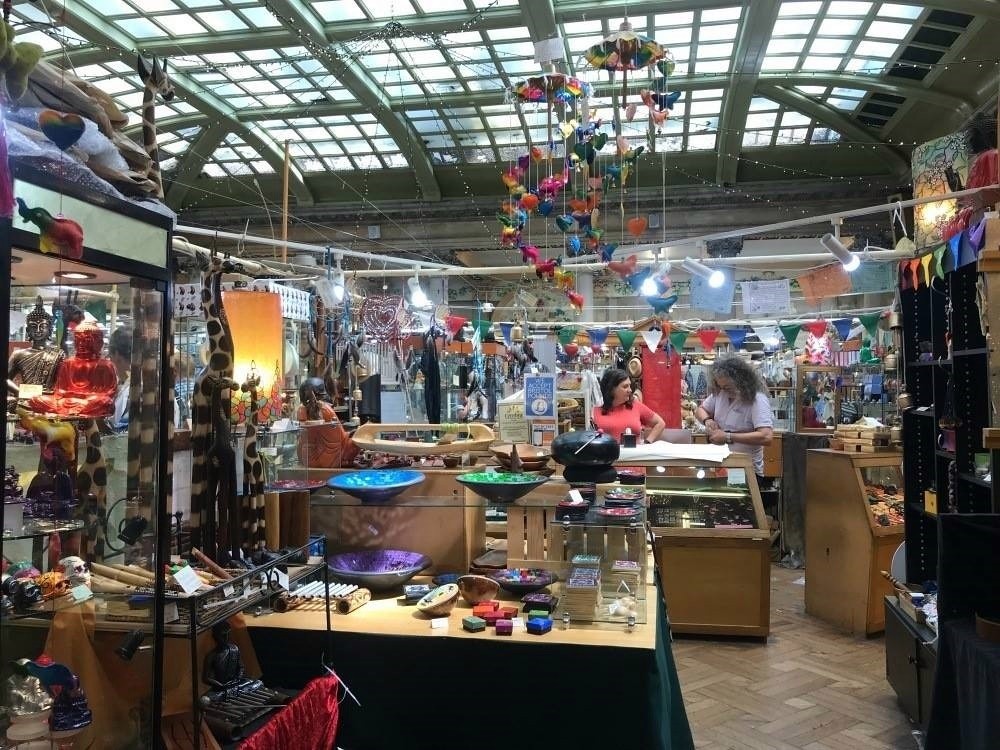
[820,217,860,272]
[407,265,428,307]
[640,248,672,295]
[681,256,725,287]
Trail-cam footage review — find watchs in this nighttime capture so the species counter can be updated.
[726,433,732,445]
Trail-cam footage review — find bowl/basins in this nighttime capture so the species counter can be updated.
[417,567,559,618]
[263,480,326,495]
[443,457,459,467]
[325,469,426,501]
[325,549,434,593]
[453,472,549,503]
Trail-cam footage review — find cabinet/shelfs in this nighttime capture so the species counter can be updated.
[0,162,1000,750]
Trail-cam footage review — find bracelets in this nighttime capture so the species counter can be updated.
[702,417,712,424]
[643,439,651,444]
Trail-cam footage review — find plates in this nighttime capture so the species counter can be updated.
[489,443,552,469]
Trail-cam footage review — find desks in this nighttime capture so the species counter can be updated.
[242,531,695,750]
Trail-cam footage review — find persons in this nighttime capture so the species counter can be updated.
[102,315,133,435]
[591,370,666,473]
[6,295,66,418]
[694,357,775,489]
[287,378,359,473]
[30,319,117,418]
